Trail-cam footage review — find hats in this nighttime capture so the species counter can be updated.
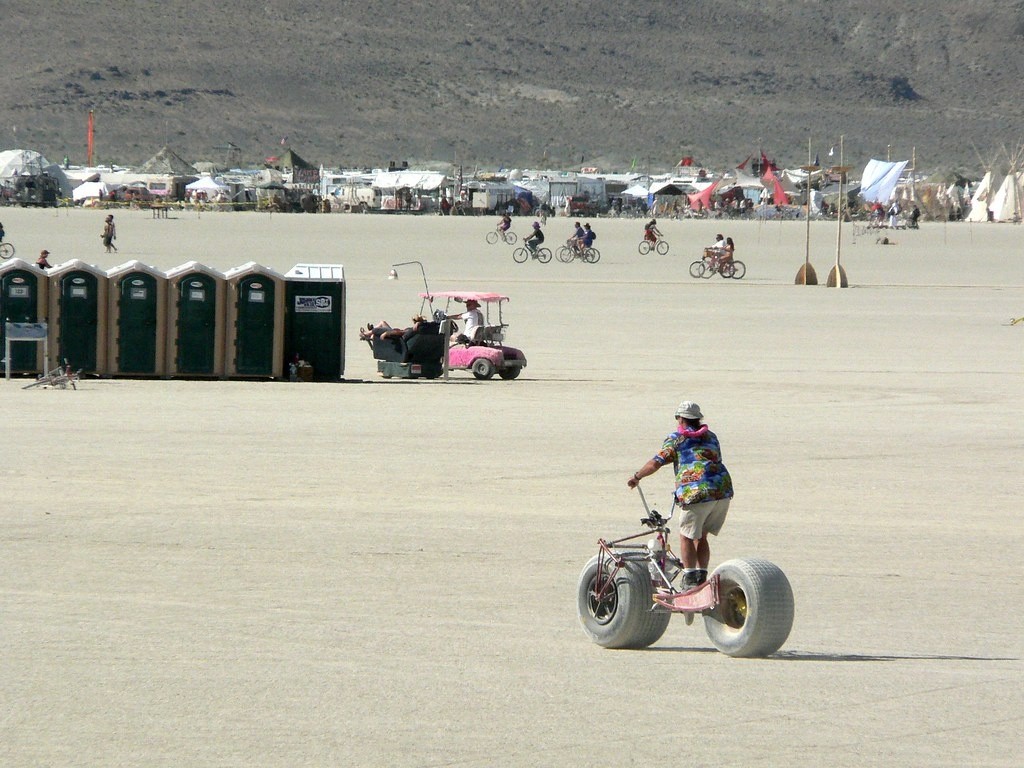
[583,223,592,227]
[532,222,540,228]
[464,300,481,308]
[675,401,704,419]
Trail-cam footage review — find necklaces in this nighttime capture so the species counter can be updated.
[678,425,708,437]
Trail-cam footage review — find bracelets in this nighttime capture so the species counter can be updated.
[634,472,640,480]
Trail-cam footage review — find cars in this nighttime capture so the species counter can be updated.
[104,186,162,202]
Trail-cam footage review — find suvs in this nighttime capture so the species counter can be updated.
[569,193,597,217]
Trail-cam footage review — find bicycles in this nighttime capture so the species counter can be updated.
[688,248,747,279]
[485,224,518,245]
[638,234,669,255]
[607,206,645,219]
[555,239,601,264]
[0,239,15,260]
[513,238,553,264]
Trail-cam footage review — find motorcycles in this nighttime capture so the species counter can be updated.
[575,481,795,658]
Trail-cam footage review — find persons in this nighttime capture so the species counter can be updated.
[627,401,734,590]
[644,219,663,250]
[36,250,51,269]
[715,197,766,214]
[872,201,919,228]
[523,222,544,257]
[448,300,483,343]
[706,233,734,272]
[360,314,424,340]
[569,222,592,252]
[496,213,511,239]
[101,215,117,253]
[99,189,117,201]
[776,200,785,211]
[183,189,207,202]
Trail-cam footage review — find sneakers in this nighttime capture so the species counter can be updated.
[680,572,698,591]
[695,570,707,586]
